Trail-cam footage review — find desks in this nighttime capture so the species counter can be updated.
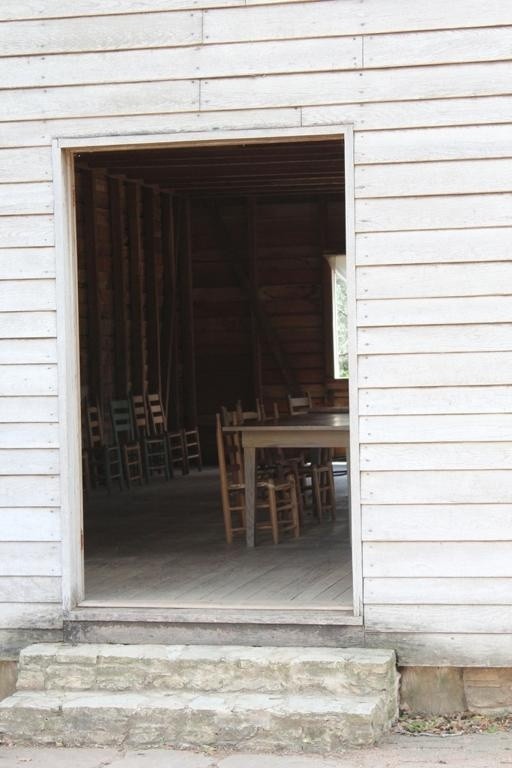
[222,413,349,548]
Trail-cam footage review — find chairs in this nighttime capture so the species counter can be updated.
[82,391,202,497]
[216,392,335,543]
